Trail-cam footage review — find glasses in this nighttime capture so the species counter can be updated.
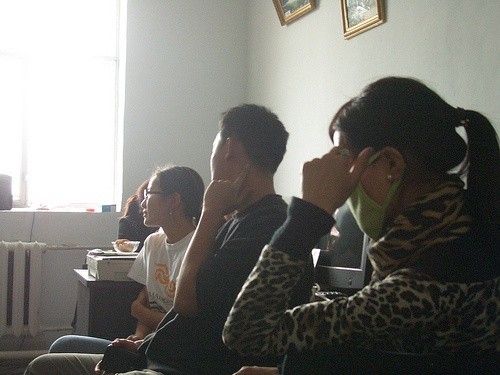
[144,189,162,195]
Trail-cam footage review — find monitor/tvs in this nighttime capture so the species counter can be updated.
[315,202,372,290]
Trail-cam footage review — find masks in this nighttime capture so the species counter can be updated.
[345,153,407,239]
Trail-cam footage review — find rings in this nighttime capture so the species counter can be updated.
[336,146,350,157]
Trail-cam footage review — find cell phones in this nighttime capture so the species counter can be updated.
[100,345,146,373]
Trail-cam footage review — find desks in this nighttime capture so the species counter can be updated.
[73,269,143,342]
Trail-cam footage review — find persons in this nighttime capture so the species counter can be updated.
[49,164,205,354]
[222,76,500,375]
[118,178,160,252]
[23,102,315,375]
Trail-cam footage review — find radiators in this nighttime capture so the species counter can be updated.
[0,240,46,337]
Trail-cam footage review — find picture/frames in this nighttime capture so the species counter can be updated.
[340,0,385,40]
[271,0,316,27]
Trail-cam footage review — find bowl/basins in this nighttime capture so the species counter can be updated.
[111,241,140,256]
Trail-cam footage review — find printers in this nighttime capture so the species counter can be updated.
[86,252,141,281]
